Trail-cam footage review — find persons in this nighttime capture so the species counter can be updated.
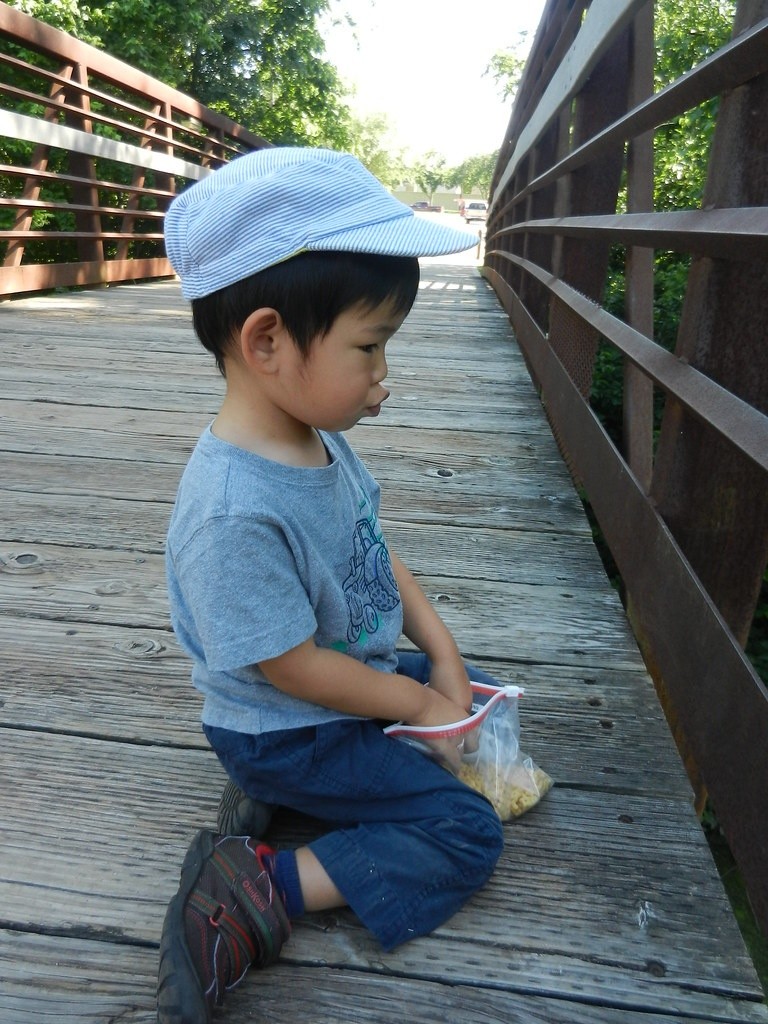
[158,148,500,1024]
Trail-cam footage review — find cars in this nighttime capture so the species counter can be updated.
[458,201,469,216]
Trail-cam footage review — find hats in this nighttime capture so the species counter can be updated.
[164,146,482,301]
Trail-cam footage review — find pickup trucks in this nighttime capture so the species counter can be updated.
[409,201,444,214]
[464,202,488,224]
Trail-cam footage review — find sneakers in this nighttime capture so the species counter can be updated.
[154,825,294,1024]
[218,774,281,845]
